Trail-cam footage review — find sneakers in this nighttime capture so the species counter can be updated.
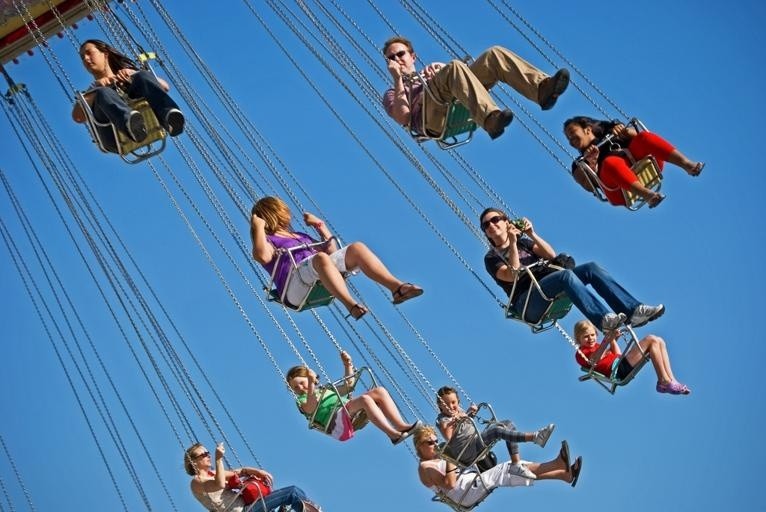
[531,423,555,449]
[628,302,666,330]
[534,67,571,111]
[481,105,515,142]
[389,419,423,446]
[600,310,629,334]
[505,461,538,481]
[124,108,148,145]
[162,107,185,138]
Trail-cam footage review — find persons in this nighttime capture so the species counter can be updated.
[435,385,553,480]
[564,116,706,208]
[252,196,424,320]
[574,319,691,395]
[72,39,184,152]
[286,352,421,445]
[414,425,583,507]
[480,206,665,336]
[383,36,570,140]
[184,443,321,512]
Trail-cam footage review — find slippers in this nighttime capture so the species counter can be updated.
[558,439,571,475]
[687,161,707,177]
[645,191,667,210]
[569,455,583,488]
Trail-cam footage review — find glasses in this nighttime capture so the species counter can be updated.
[386,48,411,60]
[425,439,438,446]
[190,451,209,461]
[480,215,506,230]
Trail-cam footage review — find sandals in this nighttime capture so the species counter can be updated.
[343,301,369,323]
[389,281,425,306]
[654,378,690,397]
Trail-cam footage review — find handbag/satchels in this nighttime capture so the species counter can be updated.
[235,476,271,506]
[528,251,576,280]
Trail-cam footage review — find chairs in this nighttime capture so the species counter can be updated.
[436,402,500,470]
[504,257,573,333]
[400,55,476,151]
[265,236,351,313]
[432,462,497,512]
[76,61,168,164]
[578,324,651,395]
[308,366,378,438]
[209,470,274,512]
[577,118,664,211]
